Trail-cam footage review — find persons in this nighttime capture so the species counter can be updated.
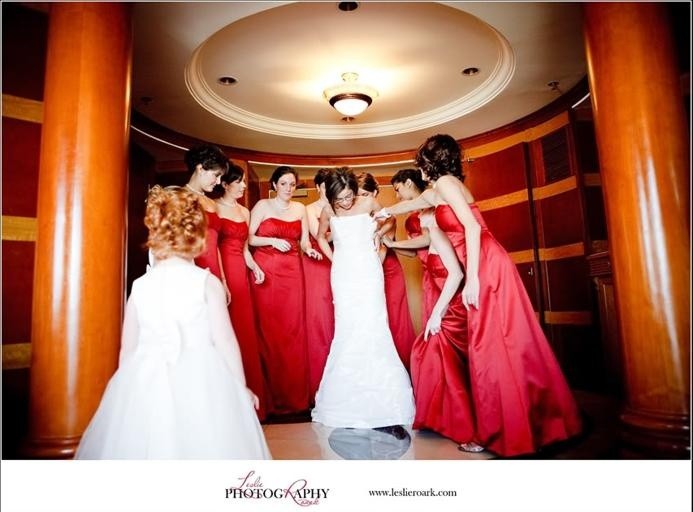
[71,132,582,460]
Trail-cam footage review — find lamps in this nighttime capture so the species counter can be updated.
[323,72,379,117]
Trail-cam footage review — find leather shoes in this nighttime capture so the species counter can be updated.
[459,442,484,453]
